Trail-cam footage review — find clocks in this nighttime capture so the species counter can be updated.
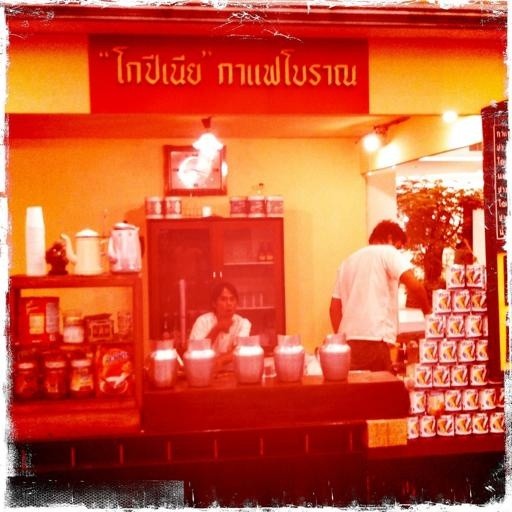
[163,144,228,199]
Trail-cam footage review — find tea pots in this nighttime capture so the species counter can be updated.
[106,220,145,272]
[59,226,105,274]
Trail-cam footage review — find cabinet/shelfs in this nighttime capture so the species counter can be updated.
[146,218,285,344]
[9,274,146,443]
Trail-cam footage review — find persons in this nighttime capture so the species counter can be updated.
[190,282,252,372]
[329,219,433,375]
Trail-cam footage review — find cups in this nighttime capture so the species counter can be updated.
[26,205,46,276]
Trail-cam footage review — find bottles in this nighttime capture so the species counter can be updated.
[494,106,508,237]
[149,333,350,389]
